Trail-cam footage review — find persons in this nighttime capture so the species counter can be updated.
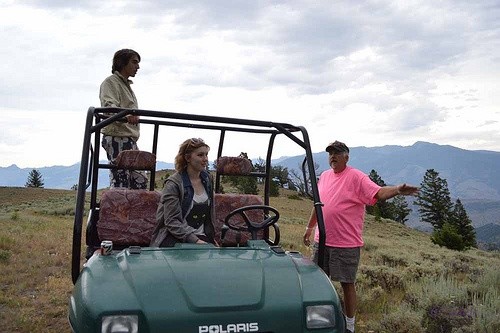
[302,140,421,333]
[149,138,219,247]
[102,48,148,189]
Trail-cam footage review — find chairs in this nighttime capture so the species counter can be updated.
[215,157,281,247]
[85,150,161,247]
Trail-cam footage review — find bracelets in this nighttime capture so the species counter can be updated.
[306,226,313,229]
[396,185,401,194]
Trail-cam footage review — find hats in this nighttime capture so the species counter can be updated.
[326,140,349,153]
